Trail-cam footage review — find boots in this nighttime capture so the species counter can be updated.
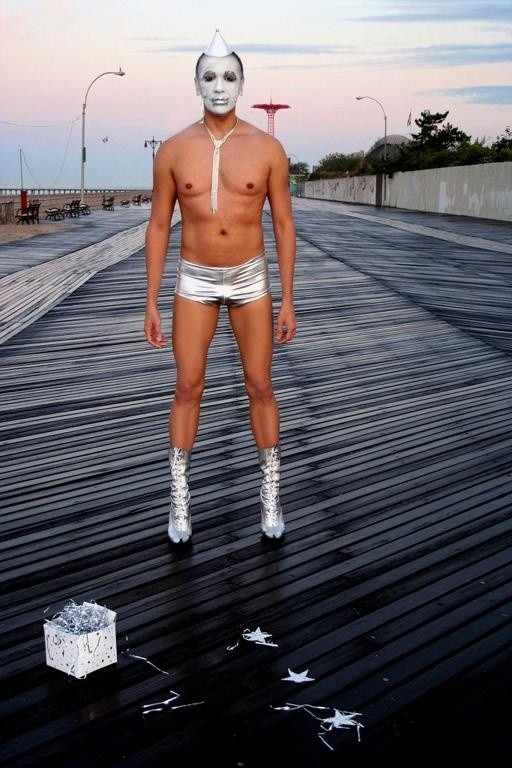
[164,445,196,544]
[258,443,286,540]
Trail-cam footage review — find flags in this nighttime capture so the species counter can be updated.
[406,112,411,129]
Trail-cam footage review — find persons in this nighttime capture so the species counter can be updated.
[143,28,297,553]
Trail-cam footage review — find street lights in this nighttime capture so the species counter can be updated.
[356,96,389,171]
[79,67,126,205]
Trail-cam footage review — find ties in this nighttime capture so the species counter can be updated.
[200,117,237,217]
